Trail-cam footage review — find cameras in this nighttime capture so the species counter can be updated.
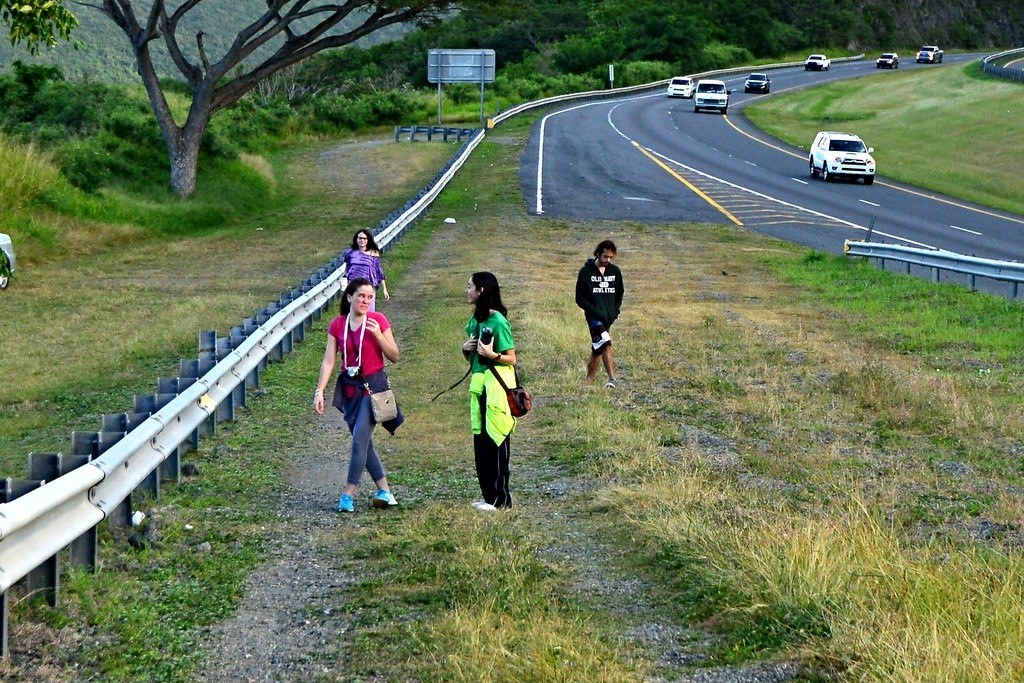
[344,367,362,380]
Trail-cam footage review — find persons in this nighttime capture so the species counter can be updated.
[313,276,401,512]
[574,240,626,389]
[461,271,517,512]
[338,228,391,312]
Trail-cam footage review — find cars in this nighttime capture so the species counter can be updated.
[667,77,695,98]
[744,73,772,94]
[875,52,899,70]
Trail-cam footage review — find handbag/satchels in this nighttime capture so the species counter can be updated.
[370,390,398,423]
[507,386,532,417]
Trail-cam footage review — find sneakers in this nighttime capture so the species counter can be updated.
[372,490,398,509]
[470,499,485,508]
[477,503,497,512]
[339,494,355,513]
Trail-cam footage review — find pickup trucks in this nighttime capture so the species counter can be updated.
[916,45,944,63]
[804,54,832,71]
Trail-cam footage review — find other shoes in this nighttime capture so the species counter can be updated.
[605,379,617,388]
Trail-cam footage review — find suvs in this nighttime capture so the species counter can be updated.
[809,131,877,185]
[693,79,732,114]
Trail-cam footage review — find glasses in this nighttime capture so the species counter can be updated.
[357,237,367,240]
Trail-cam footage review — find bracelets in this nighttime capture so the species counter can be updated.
[315,390,324,396]
[493,353,501,361]
